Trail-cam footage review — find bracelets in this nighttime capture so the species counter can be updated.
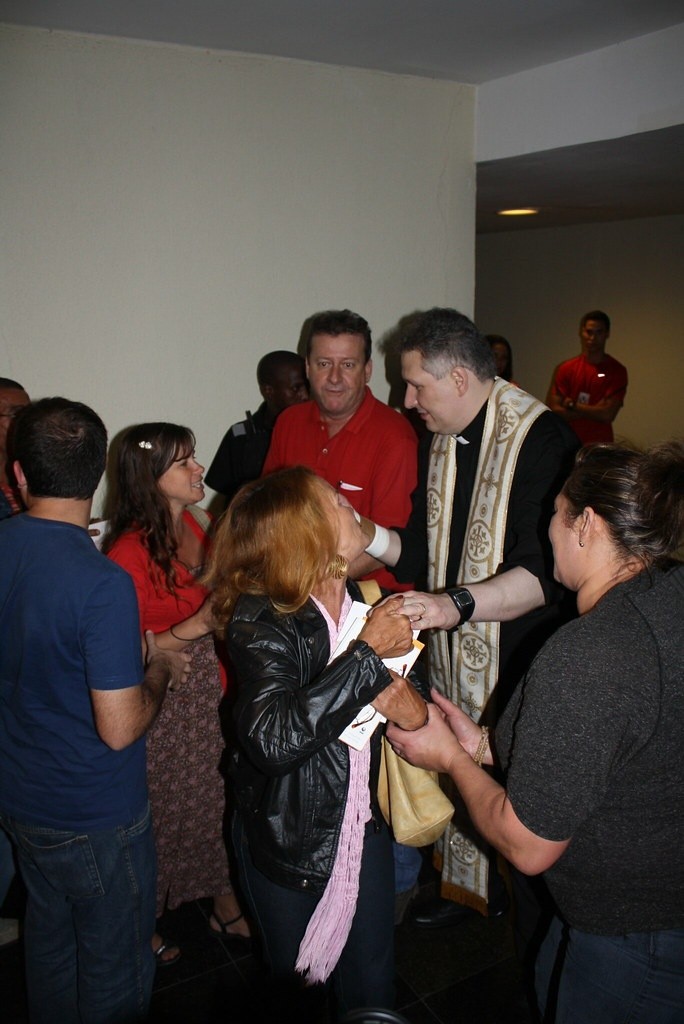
[474,726,489,766]
[171,627,195,642]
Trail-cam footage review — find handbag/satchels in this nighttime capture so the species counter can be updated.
[357,578,457,848]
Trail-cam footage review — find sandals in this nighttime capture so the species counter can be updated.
[155,938,182,967]
[208,910,258,943]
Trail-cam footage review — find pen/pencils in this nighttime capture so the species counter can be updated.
[336,480,342,491]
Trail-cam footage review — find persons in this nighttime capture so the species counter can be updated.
[259,309,419,595]
[486,335,521,389]
[0,377,192,1024]
[546,310,629,447]
[205,467,427,1024]
[353,308,576,1024]
[105,422,257,966]
[385,440,684,1024]
[204,350,311,499]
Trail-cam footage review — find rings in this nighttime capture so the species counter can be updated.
[420,603,426,615]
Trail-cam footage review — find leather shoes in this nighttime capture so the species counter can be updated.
[409,886,509,928]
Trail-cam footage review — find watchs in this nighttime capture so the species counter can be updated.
[567,401,575,408]
[446,587,475,633]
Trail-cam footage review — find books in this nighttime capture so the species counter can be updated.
[326,601,425,751]
